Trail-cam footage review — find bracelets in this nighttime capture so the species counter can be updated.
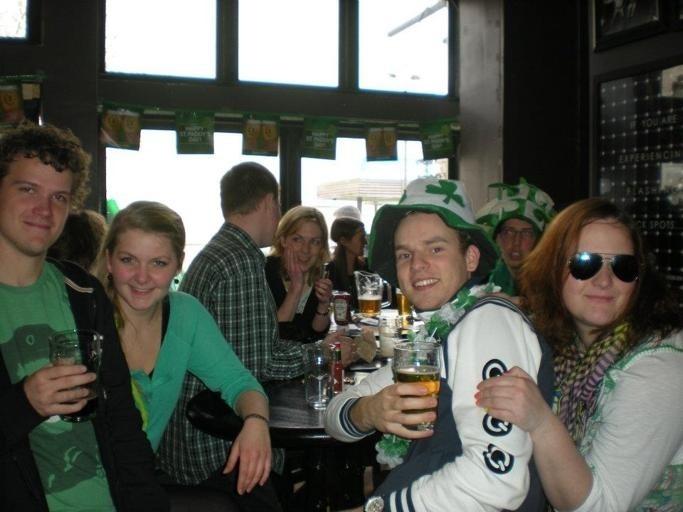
[313,309,330,316]
[243,413,270,424]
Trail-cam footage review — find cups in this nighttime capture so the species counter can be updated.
[392,339,443,436]
[332,294,352,326]
[394,287,413,316]
[378,316,403,360]
[353,270,392,318]
[47,328,110,425]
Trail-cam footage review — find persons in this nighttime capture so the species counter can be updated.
[47,203,110,278]
[467,179,560,322]
[329,204,360,219]
[0,123,167,510]
[322,214,399,311]
[149,161,355,512]
[318,175,556,512]
[97,201,284,511]
[259,204,335,337]
[468,196,683,512]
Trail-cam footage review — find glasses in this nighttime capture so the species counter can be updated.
[498,226,538,240]
[566,249,640,283]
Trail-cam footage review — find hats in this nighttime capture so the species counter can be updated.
[366,177,504,290]
[474,177,561,239]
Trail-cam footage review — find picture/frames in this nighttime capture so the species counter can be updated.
[592,1,671,54]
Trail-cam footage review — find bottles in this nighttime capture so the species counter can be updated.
[305,339,335,410]
[319,262,330,279]
[331,341,345,394]
